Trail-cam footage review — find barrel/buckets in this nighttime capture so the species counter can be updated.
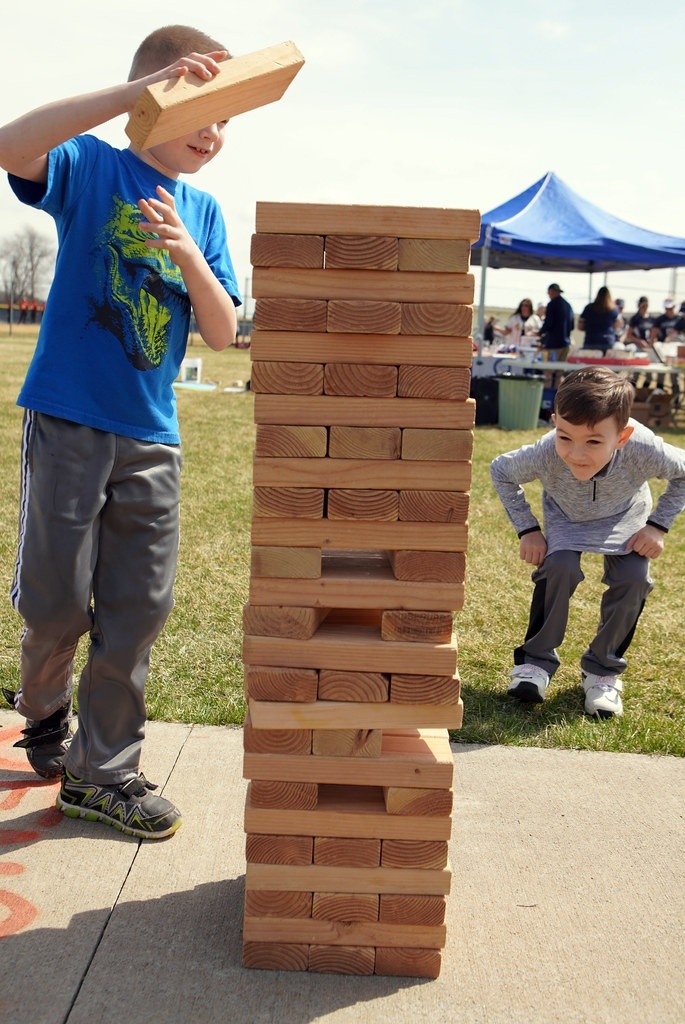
[498,375,546,432]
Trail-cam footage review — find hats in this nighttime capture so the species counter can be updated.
[664,297,676,309]
[615,298,625,308]
[549,284,563,292]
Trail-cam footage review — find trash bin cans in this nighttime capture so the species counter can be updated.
[488,373,545,430]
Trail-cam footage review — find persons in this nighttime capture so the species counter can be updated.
[482,283,684,408]
[489,364,684,719]
[0,23,240,842]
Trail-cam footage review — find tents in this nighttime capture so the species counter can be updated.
[469,170,684,331]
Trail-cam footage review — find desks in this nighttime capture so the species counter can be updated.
[499,353,685,387]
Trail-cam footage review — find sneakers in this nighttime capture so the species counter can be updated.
[55,765,182,838]
[12,693,73,778]
[507,664,549,702]
[581,669,623,720]
